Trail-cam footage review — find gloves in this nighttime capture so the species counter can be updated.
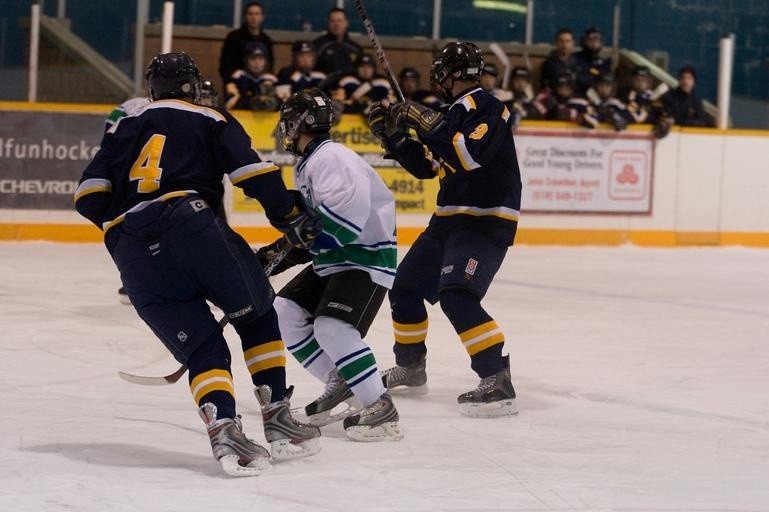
[603,101,629,133]
[652,112,673,140]
[368,101,406,148]
[266,187,324,252]
[387,96,447,141]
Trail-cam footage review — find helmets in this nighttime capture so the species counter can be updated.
[355,54,377,69]
[593,71,616,87]
[278,87,335,133]
[510,66,530,82]
[579,28,603,54]
[631,65,650,78]
[252,384,321,442]
[290,40,313,54]
[550,72,575,105]
[399,67,420,80]
[239,39,270,62]
[430,53,443,85]
[436,40,484,85]
[146,49,201,99]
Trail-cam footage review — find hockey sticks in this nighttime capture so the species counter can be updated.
[120,209,315,385]
[490,42,511,90]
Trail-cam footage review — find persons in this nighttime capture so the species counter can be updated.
[219,0,439,113]
[484,29,708,136]
[73,52,324,468]
[250,87,400,432]
[368,40,523,404]
[100,81,237,297]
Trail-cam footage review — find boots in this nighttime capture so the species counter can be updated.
[456,353,517,406]
[375,355,430,389]
[342,391,400,431]
[305,367,354,417]
[197,401,271,463]
